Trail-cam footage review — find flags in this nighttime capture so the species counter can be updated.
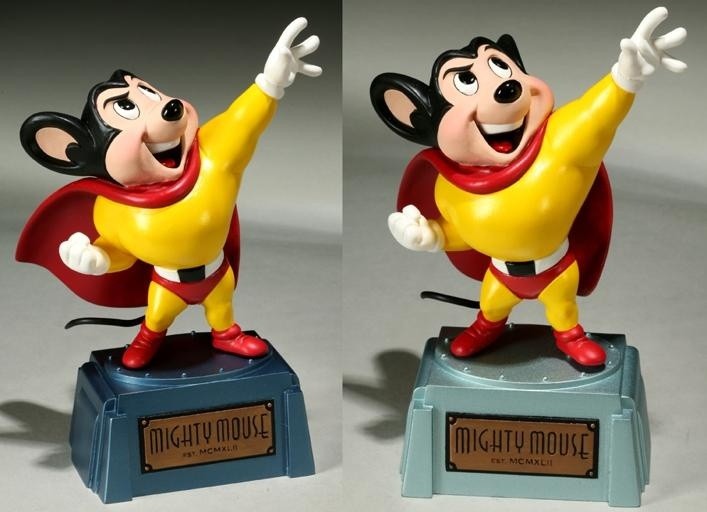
[15,16,325,503]
[366,6,689,509]
